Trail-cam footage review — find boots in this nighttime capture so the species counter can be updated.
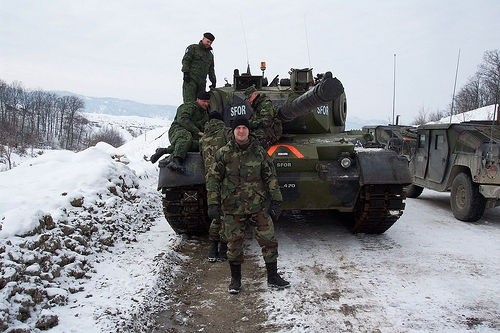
[208,240,218,262]
[217,242,228,262]
[151,147,168,164]
[264,261,290,288]
[227,263,242,293]
[167,156,185,173]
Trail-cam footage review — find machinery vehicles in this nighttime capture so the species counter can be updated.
[159,70,412,238]
[360,115,500,223]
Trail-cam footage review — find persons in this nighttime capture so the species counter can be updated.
[205,117,291,294]
[181,33,216,103]
[150,92,209,169]
[244,86,283,149]
[197,111,234,262]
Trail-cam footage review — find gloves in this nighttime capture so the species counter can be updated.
[208,204,219,219]
[183,72,190,82]
[209,82,216,88]
[270,200,282,220]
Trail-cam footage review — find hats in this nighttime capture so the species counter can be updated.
[244,86,257,98]
[209,110,224,122]
[231,114,249,133]
[204,32,215,41]
[197,91,211,100]
